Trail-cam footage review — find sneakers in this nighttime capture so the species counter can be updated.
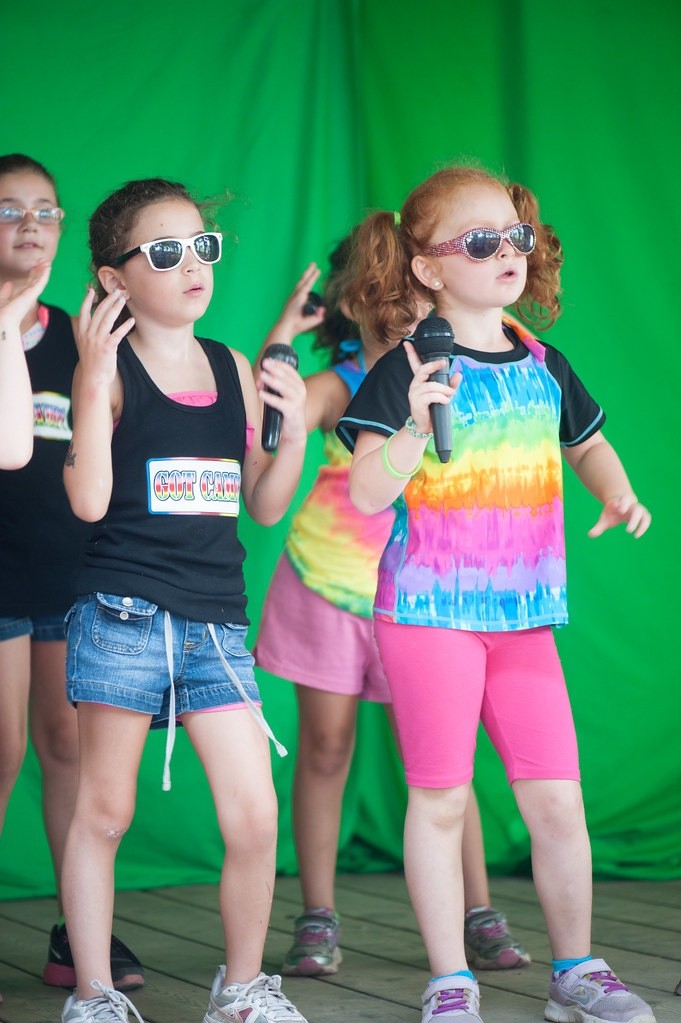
[280,907,343,974]
[463,906,531,970]
[543,958,655,1023]
[422,970,482,1022]
[60,980,144,1023]
[43,924,145,989]
[203,965,308,1023]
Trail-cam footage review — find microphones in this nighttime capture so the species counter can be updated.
[415,318,453,462]
[261,343,298,451]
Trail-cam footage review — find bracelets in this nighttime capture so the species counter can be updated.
[405,414,435,439]
[382,431,424,478]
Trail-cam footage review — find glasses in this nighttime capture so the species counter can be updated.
[421,223,536,262]
[0,206,65,224]
[111,232,223,271]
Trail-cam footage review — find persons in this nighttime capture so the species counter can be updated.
[61,177,306,1023]
[251,226,529,978]
[333,170,652,1023]
[0,154,144,989]
